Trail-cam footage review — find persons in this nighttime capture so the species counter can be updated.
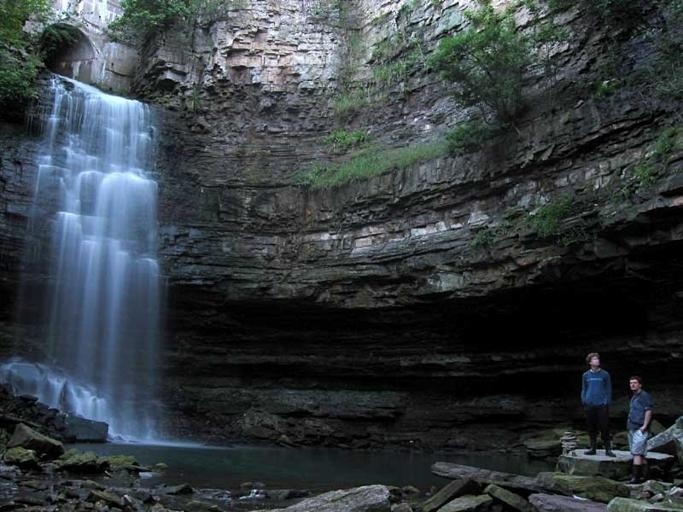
[625,375,652,484]
[580,352,616,458]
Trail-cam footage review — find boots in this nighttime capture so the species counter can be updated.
[582,438,616,457]
[625,462,649,485]
